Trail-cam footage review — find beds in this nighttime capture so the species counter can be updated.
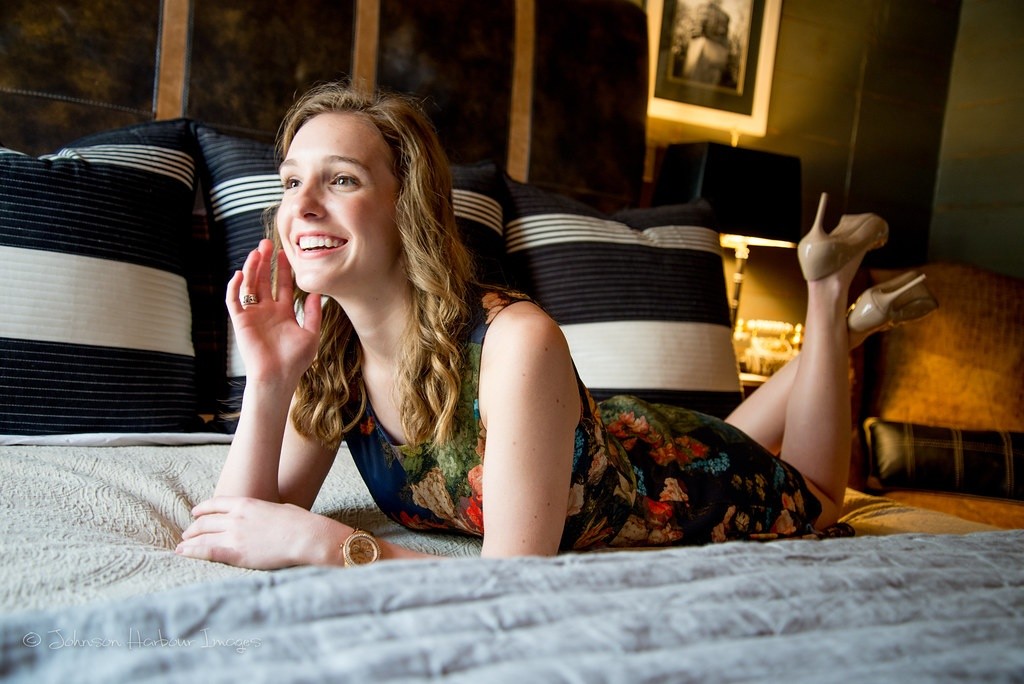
[0,124,1024,684]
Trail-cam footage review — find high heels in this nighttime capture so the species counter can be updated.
[847,270,937,332]
[797,193,888,281]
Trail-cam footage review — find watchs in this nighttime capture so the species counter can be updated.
[339,527,382,570]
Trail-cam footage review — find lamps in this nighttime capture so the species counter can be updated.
[652,142,811,338]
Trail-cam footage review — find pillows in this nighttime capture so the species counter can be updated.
[504,174,742,418]
[197,123,507,442]
[0,119,208,435]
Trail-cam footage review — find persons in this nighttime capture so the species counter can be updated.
[169,80,941,572]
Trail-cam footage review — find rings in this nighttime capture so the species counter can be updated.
[241,294,260,306]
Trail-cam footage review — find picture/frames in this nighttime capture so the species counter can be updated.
[644,0,782,137]
